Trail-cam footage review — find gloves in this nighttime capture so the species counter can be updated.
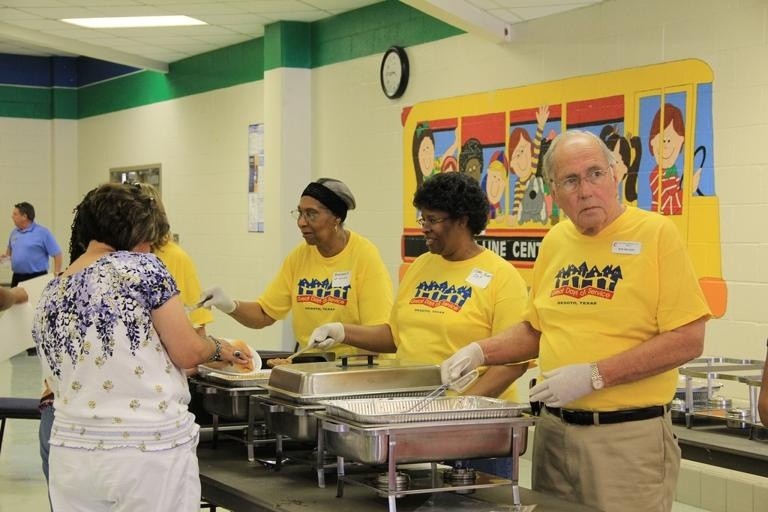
[200,285,236,314]
[441,342,484,391]
[309,322,345,350]
[529,363,594,408]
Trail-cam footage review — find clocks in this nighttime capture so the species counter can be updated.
[379,45,411,102]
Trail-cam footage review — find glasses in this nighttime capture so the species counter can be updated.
[417,215,450,226]
[553,168,607,193]
[290,208,318,221]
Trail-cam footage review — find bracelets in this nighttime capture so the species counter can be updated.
[206,334,222,363]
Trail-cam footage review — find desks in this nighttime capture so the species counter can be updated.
[196,428,604,511]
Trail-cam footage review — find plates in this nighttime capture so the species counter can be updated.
[203,337,261,377]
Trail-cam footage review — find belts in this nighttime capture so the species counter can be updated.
[544,400,673,425]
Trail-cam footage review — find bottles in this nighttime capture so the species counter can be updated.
[725,406,754,430]
[671,395,686,422]
[706,393,732,408]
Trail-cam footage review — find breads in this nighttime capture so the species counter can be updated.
[207,361,229,370]
[231,339,255,374]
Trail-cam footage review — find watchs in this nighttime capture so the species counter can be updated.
[589,362,604,390]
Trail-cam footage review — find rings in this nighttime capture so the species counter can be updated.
[234,350,241,357]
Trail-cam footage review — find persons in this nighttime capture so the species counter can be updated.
[307,172,536,480]
[440,129,712,512]
[199,178,394,360]
[38,185,214,511]
[33,179,253,512]
[413,102,702,226]
[1,201,61,356]
[1,287,28,311]
